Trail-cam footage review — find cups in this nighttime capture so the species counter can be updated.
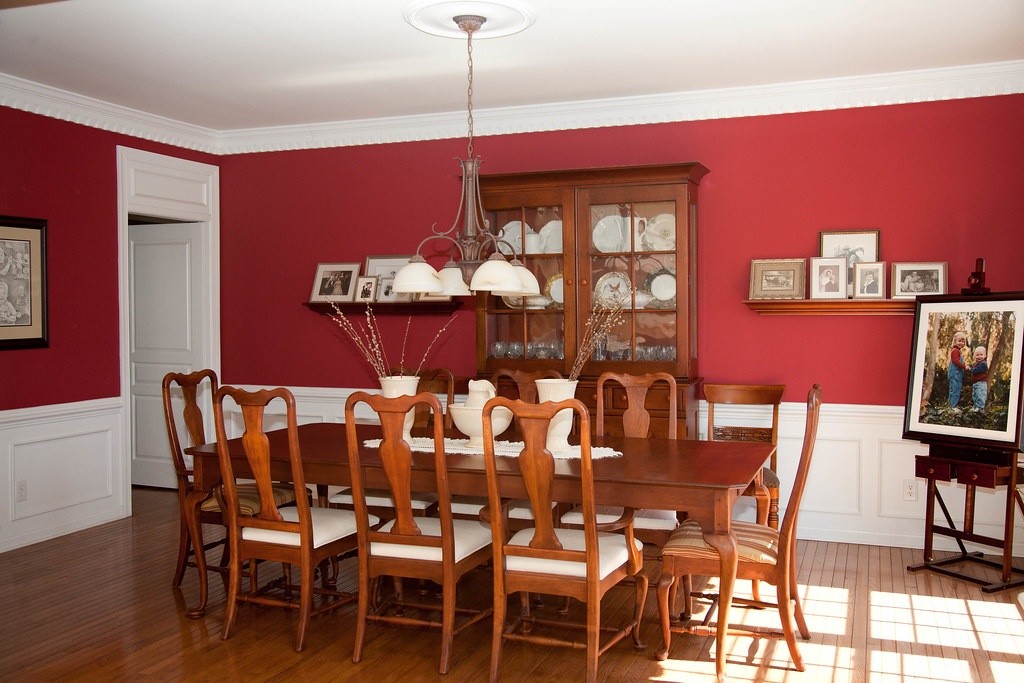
[490,338,565,360]
[591,334,677,362]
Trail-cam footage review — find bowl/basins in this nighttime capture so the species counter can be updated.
[449,404,514,447]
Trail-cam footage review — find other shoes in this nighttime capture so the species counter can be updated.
[969,406,984,414]
[947,406,963,414]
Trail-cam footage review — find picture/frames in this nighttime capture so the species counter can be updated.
[0,215,50,350]
[310,261,362,301]
[364,252,424,299]
[354,274,379,303]
[817,226,880,299]
[416,291,452,301]
[891,260,949,299]
[379,278,415,301]
[902,290,1024,452]
[854,261,887,297]
[810,256,850,297]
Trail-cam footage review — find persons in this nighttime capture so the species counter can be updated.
[361,286,368,298]
[948,332,971,414]
[324,272,347,295]
[0,240,28,325]
[834,244,864,265]
[819,269,839,292]
[901,270,936,292]
[384,286,390,296]
[969,346,989,411]
[863,271,878,293]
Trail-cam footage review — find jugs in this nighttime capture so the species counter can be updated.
[515,229,549,253]
[465,379,497,406]
[612,215,647,252]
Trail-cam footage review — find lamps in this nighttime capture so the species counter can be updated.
[394,0,540,298]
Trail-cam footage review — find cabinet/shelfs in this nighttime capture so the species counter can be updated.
[749,257,806,300]
[448,162,710,440]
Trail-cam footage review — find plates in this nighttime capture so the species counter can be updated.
[498,221,532,255]
[592,215,624,253]
[503,297,522,309]
[644,268,677,310]
[544,273,564,309]
[644,213,676,251]
[594,273,633,308]
[539,220,562,253]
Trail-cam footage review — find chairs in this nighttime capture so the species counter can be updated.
[212,386,381,653]
[653,379,824,670]
[482,397,649,682]
[554,369,692,622]
[344,390,509,675]
[323,370,456,600]
[436,367,566,634]
[697,384,787,529]
[163,368,316,587]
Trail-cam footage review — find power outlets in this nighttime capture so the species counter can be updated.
[902,480,920,501]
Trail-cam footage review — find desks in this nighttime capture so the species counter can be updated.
[183,419,782,683]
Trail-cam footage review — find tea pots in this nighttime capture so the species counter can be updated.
[615,287,657,309]
[513,294,553,310]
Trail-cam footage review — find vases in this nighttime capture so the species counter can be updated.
[535,376,579,450]
[374,374,422,444]
